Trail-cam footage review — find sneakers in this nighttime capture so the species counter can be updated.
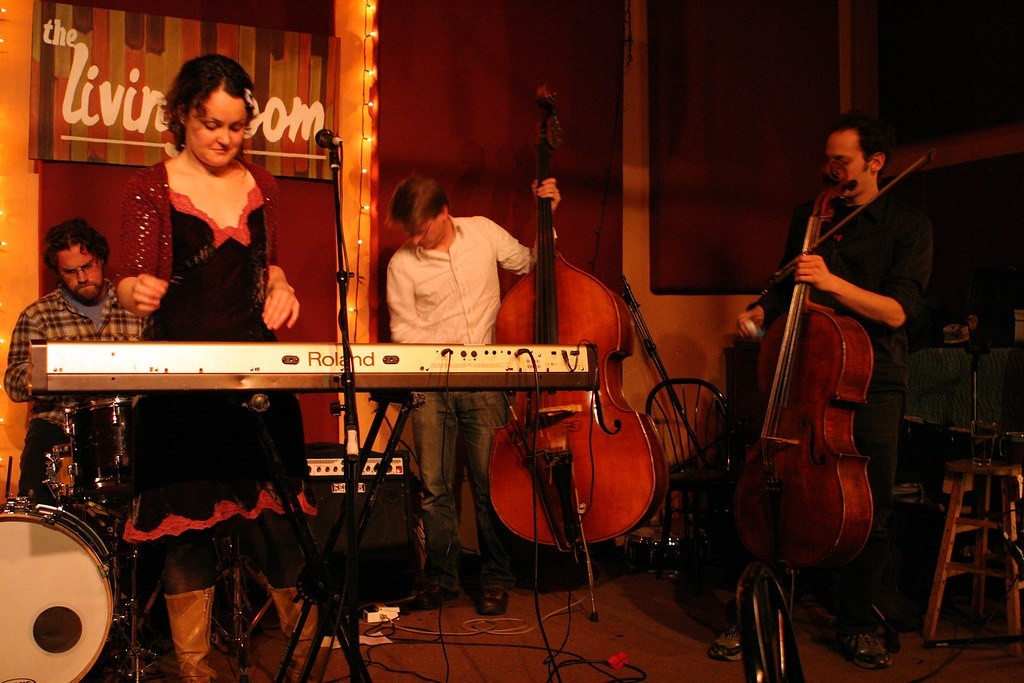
[477,586,509,614]
[709,623,744,661]
[414,584,458,609]
[843,632,892,669]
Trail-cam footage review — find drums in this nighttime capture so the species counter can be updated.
[64,396,133,496]
[49,444,76,497]
[0,498,117,683]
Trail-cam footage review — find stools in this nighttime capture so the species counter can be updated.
[924,456,1024,660]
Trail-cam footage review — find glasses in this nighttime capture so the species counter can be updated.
[59,257,99,279]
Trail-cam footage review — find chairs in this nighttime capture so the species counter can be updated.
[645,376,730,579]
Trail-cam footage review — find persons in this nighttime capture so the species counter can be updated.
[708,109,934,669]
[114,53,319,683]
[386,173,561,615]
[5,218,166,655]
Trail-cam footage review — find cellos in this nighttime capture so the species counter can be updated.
[484,82,670,624]
[730,154,878,620]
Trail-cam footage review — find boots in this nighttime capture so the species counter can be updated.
[163,585,218,683]
[267,584,319,683]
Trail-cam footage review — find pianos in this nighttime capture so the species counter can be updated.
[26,338,603,681]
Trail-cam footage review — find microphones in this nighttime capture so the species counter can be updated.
[315,127,346,150]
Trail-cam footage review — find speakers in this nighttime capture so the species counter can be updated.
[302,440,422,609]
